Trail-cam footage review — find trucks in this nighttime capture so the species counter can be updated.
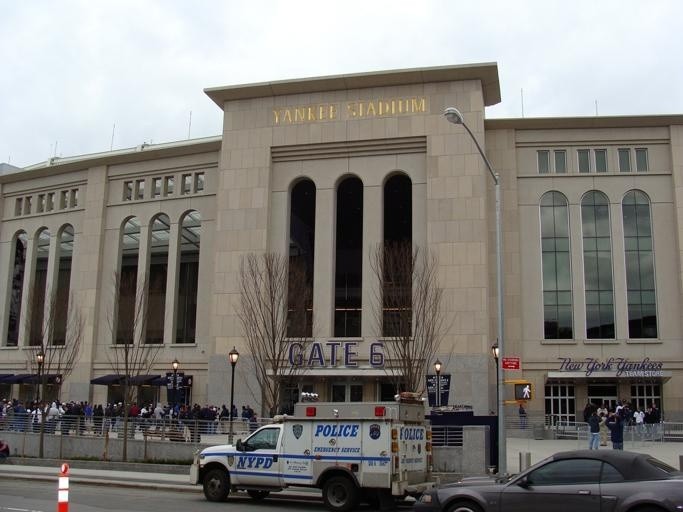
[189,399,440,509]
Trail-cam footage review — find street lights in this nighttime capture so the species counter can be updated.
[442,106,509,482]
[33,347,45,424]
[171,356,180,409]
[491,338,499,419]
[433,358,443,407]
[228,345,240,444]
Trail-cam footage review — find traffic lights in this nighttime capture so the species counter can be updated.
[514,381,535,402]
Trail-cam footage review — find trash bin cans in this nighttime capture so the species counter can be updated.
[520,452,531,475]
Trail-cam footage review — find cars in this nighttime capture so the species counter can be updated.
[418,449,682,512]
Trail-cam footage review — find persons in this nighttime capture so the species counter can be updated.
[0,394,258,443]
[582,399,661,450]
[518,403,528,431]
